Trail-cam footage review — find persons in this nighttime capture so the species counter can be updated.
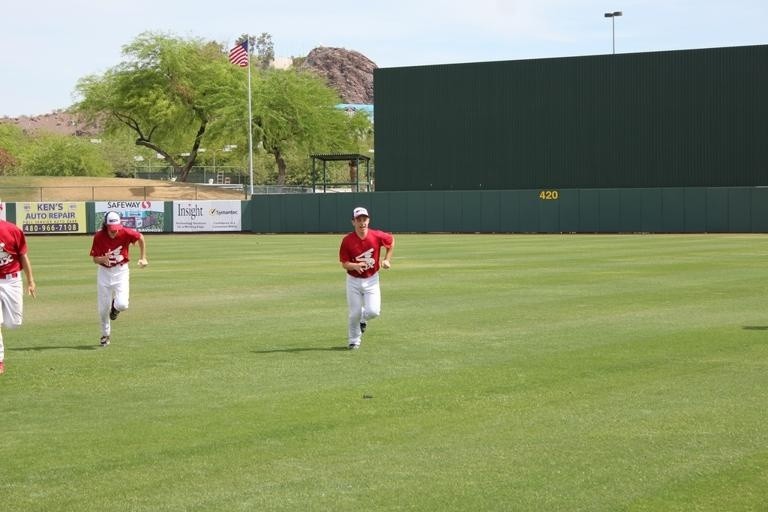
[1,201,38,376]
[88,211,149,345]
[339,207,396,350]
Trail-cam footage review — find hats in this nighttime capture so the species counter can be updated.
[352,206,370,219]
[105,211,123,231]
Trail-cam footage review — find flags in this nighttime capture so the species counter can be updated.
[228,41,249,67]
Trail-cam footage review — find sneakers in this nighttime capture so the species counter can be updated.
[360,318,368,333]
[0,362,5,374]
[110,299,120,320]
[349,344,360,352]
[100,336,111,346]
